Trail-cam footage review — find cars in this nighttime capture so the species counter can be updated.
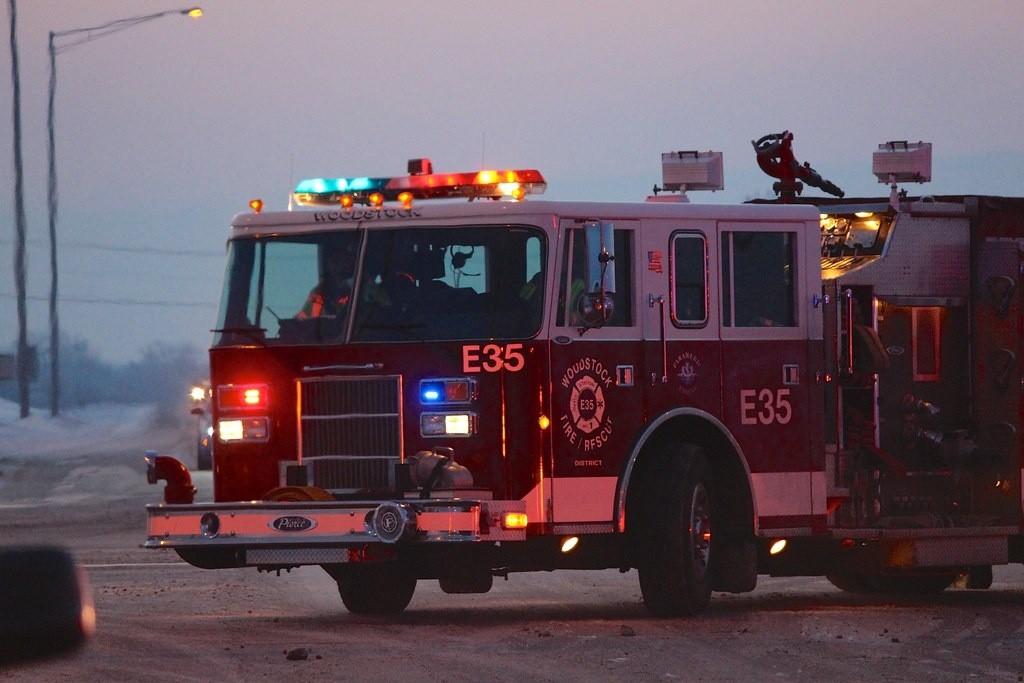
[191,377,213,471]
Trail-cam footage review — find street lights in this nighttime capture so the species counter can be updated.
[51,5,201,415]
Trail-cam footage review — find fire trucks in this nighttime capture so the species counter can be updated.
[137,129,1023,620]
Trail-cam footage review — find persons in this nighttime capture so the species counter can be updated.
[297,248,590,339]
